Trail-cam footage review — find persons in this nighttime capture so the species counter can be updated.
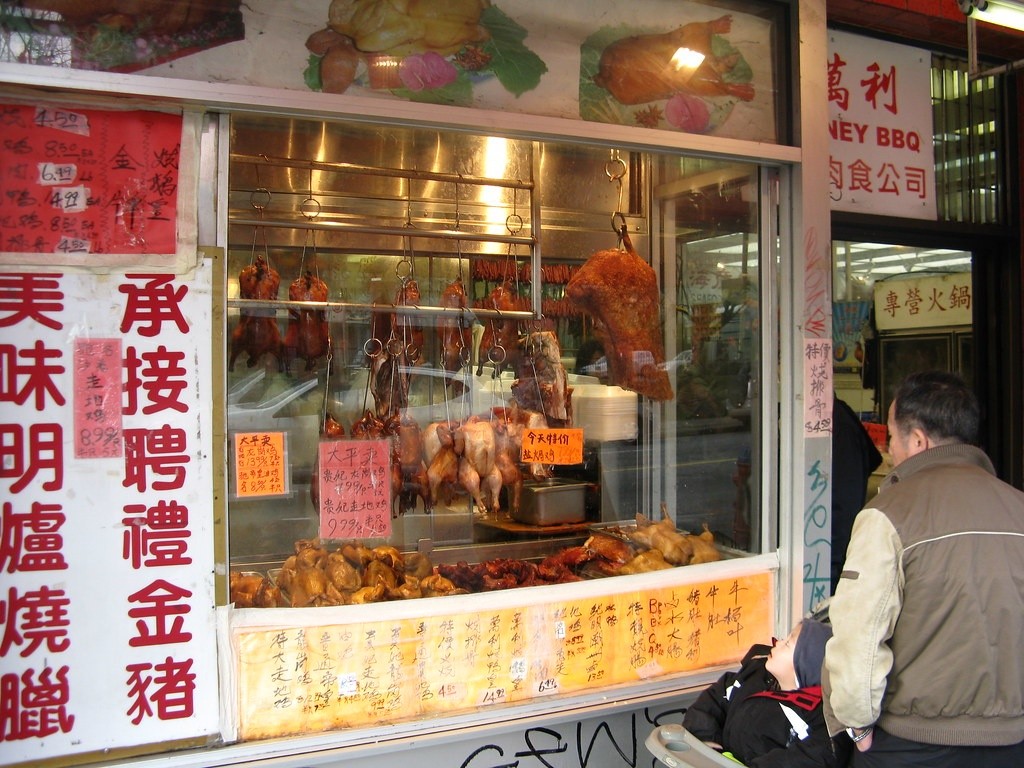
[555,340,609,482]
[831,388,881,598]
[681,617,842,768]
[823,372,1023,768]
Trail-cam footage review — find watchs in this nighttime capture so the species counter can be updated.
[846,726,872,742]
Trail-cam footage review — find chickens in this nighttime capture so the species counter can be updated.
[228,257,555,520]
[594,11,757,104]
[326,0,491,53]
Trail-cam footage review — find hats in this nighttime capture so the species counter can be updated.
[792,617,834,688]
